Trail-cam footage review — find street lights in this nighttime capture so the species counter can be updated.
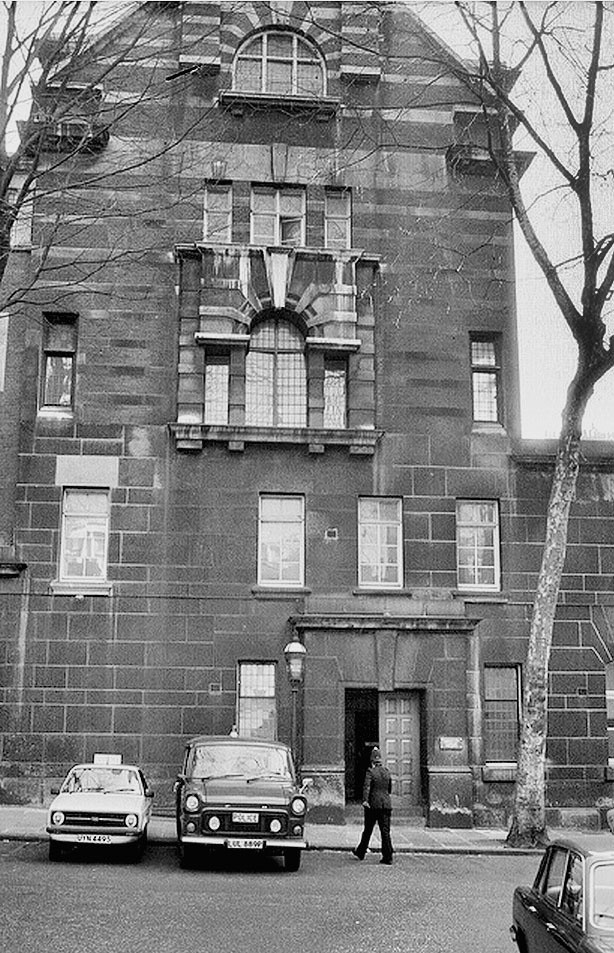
[283,627,308,788]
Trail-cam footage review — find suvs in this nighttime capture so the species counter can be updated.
[171,734,315,874]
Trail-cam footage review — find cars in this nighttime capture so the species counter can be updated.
[45,762,155,864]
[510,835,614,953]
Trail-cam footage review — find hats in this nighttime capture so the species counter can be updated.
[370,749,382,762]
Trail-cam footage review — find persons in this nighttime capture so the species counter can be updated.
[351,746,394,865]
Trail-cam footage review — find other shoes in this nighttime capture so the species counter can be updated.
[351,847,365,861]
[380,858,393,865]
